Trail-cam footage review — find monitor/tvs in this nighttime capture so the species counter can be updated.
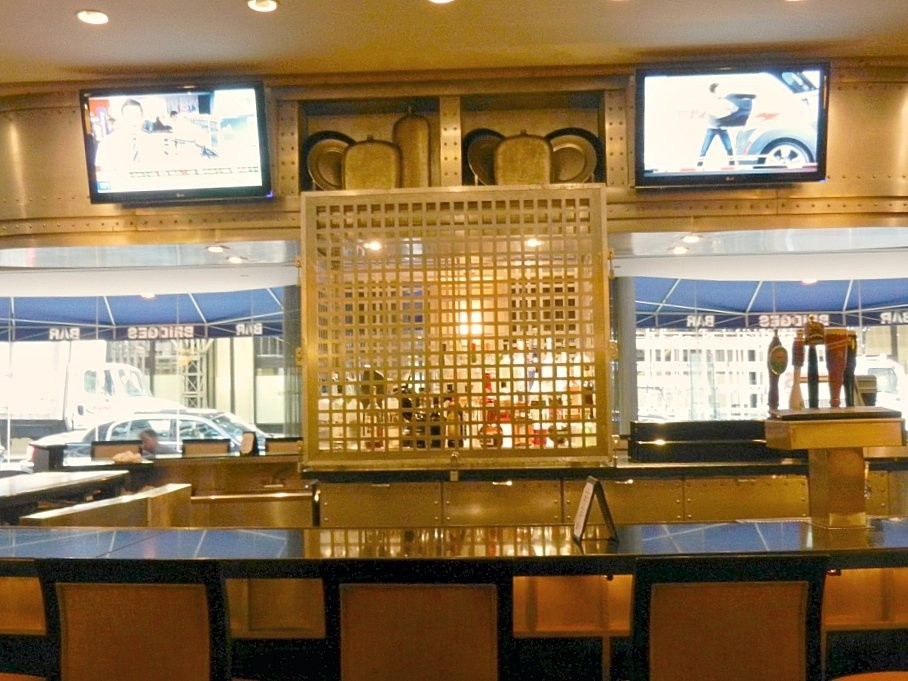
[635,61,830,186]
[79,80,270,205]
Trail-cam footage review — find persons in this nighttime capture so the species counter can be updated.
[93,99,193,191]
[138,428,181,458]
[697,84,739,168]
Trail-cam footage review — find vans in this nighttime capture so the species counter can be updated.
[776,356,907,419]
[10,362,185,422]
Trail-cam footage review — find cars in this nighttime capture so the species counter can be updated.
[734,89,819,170]
[24,407,273,458]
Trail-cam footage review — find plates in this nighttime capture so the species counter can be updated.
[543,127,603,183]
[301,131,357,190]
[462,129,506,185]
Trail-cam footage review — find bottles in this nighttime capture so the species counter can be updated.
[441,337,590,398]
[382,377,403,448]
[444,384,455,420]
[415,388,427,448]
[479,399,503,448]
[530,400,547,446]
[318,377,365,451]
[583,365,593,405]
[364,386,384,449]
[499,381,511,416]
[431,397,445,447]
[447,397,464,447]
[549,389,563,422]
[514,392,534,449]
[564,368,581,421]
